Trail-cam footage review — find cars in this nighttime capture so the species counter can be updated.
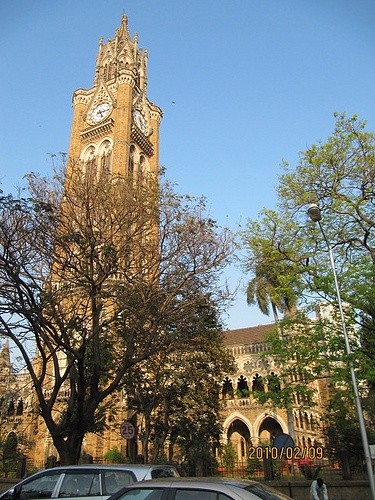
[106,477,294,500]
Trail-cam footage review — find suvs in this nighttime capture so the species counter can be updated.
[1,463,180,499]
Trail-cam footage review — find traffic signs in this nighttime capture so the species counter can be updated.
[119,420,135,442]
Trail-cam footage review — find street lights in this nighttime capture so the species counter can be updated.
[305,204,375,496]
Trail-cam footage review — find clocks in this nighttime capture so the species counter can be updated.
[132,108,148,136]
[90,101,111,123]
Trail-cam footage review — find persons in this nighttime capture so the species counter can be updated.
[309,478,328,500]
